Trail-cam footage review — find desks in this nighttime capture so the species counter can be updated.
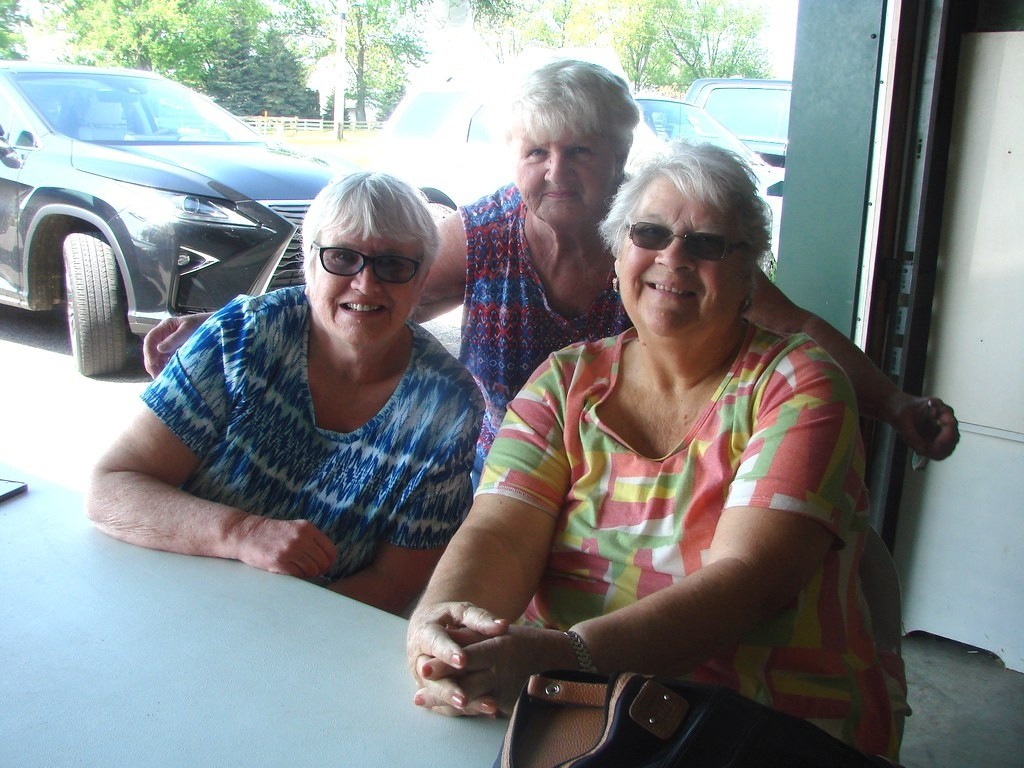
[0,472,513,768]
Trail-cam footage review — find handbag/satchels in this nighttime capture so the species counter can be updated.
[492,670,905,768]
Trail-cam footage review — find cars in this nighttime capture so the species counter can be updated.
[628,93,784,264]
[1,57,340,378]
[667,78,793,170]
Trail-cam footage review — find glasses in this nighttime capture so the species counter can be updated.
[625,222,749,261]
[312,241,422,285]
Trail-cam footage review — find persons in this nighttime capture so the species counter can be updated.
[85,172,486,616]
[406,142,908,764]
[144,60,960,493]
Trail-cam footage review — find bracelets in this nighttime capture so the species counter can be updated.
[563,631,596,674]
